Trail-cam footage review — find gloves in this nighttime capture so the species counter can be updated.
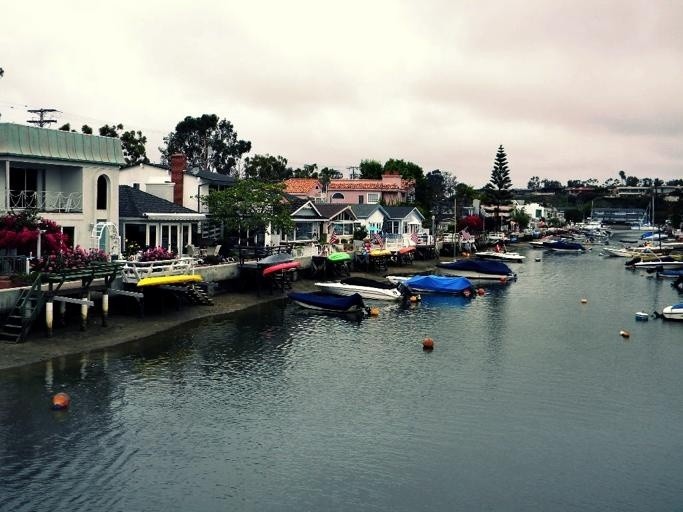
[605,231,682,279]
[662,305,683,320]
[313,282,405,302]
[435,261,516,280]
[370,250,392,256]
[399,247,416,255]
[136,274,204,286]
[286,291,362,313]
[327,253,353,261]
[387,276,468,295]
[474,252,526,260]
[528,241,593,254]
[256,253,301,277]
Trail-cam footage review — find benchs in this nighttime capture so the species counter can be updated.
[328,233,337,244]
[462,228,470,239]
[411,233,418,244]
